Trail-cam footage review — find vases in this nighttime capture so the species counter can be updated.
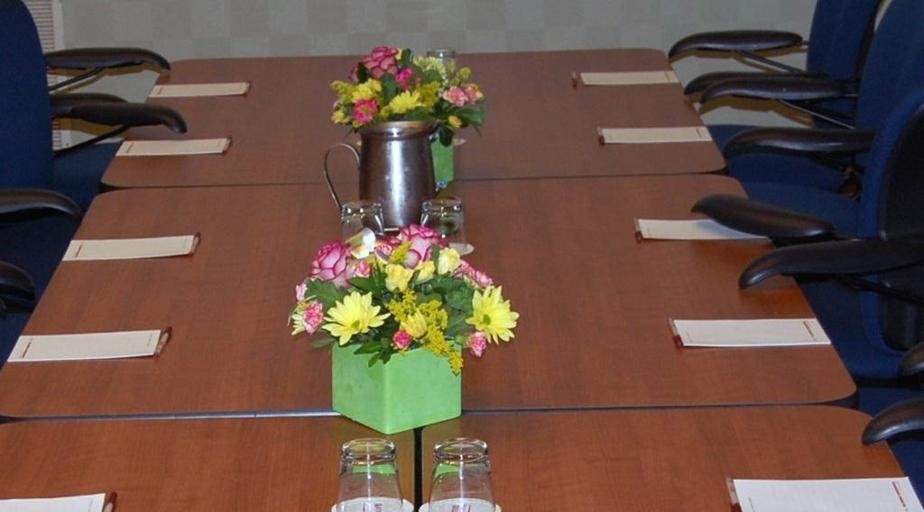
[330,340,466,436]
[353,117,443,236]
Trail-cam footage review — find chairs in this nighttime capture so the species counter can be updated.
[667,1,921,512]
[0,1,188,372]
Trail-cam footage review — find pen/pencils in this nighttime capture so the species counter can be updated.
[244,80,251,94]
[156,326,172,355]
[103,492,117,512]
[596,124,605,146]
[632,218,642,242]
[725,476,741,512]
[191,232,201,254]
[222,135,232,155]
[667,317,683,349]
[571,71,578,88]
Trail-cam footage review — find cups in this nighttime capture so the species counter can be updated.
[419,197,468,257]
[426,46,457,69]
[339,201,383,243]
[426,435,497,510]
[334,437,401,510]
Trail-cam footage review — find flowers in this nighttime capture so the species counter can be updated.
[329,42,488,150]
[287,218,518,378]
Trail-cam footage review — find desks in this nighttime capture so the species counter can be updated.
[6,418,413,512]
[412,401,924,512]
[101,53,365,189]
[446,172,859,405]
[448,48,731,173]
[2,186,357,408]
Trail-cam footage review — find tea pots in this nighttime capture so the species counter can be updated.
[318,120,435,232]
